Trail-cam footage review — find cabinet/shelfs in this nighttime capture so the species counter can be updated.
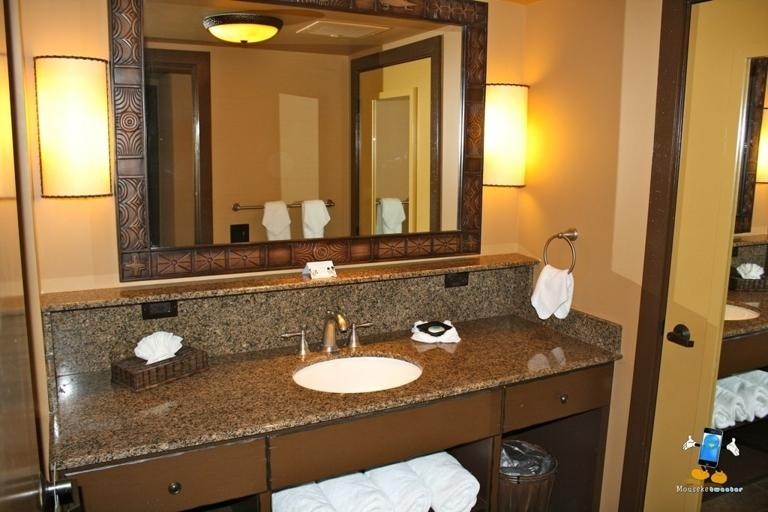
[499,360,615,511]
[66,434,270,511]
[267,386,503,510]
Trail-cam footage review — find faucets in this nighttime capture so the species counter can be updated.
[318,310,348,352]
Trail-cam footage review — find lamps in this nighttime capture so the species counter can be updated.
[203,13,281,45]
[483,82,531,188]
[34,54,115,198]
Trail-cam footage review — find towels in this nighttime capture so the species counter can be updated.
[364,461,433,511]
[262,200,291,240]
[318,472,394,511]
[532,265,575,323]
[407,451,481,511]
[376,198,407,233]
[413,319,456,344]
[717,374,768,418]
[715,384,749,423]
[736,367,766,390]
[270,480,335,511]
[708,403,735,430]
[300,198,331,239]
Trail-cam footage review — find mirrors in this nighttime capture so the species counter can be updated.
[107,0,489,282]
[351,33,446,237]
[615,0,767,510]
[733,57,767,234]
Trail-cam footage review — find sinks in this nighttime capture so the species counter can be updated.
[292,351,424,393]
[724,299,762,321]
